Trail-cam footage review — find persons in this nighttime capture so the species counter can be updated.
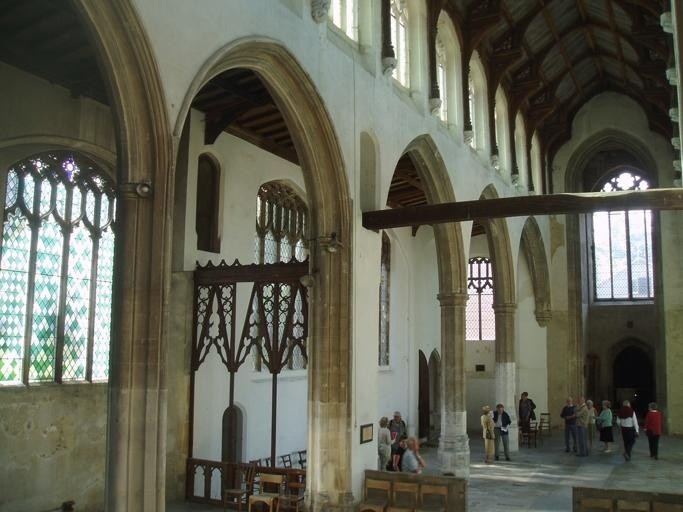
[481,405,495,464]
[560,396,616,457]
[519,392,536,430]
[492,404,511,461]
[617,400,640,460]
[378,411,426,474]
[643,402,662,460]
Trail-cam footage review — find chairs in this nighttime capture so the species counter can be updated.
[223,450,449,512]
[579,497,683,512]
[522,413,551,448]
